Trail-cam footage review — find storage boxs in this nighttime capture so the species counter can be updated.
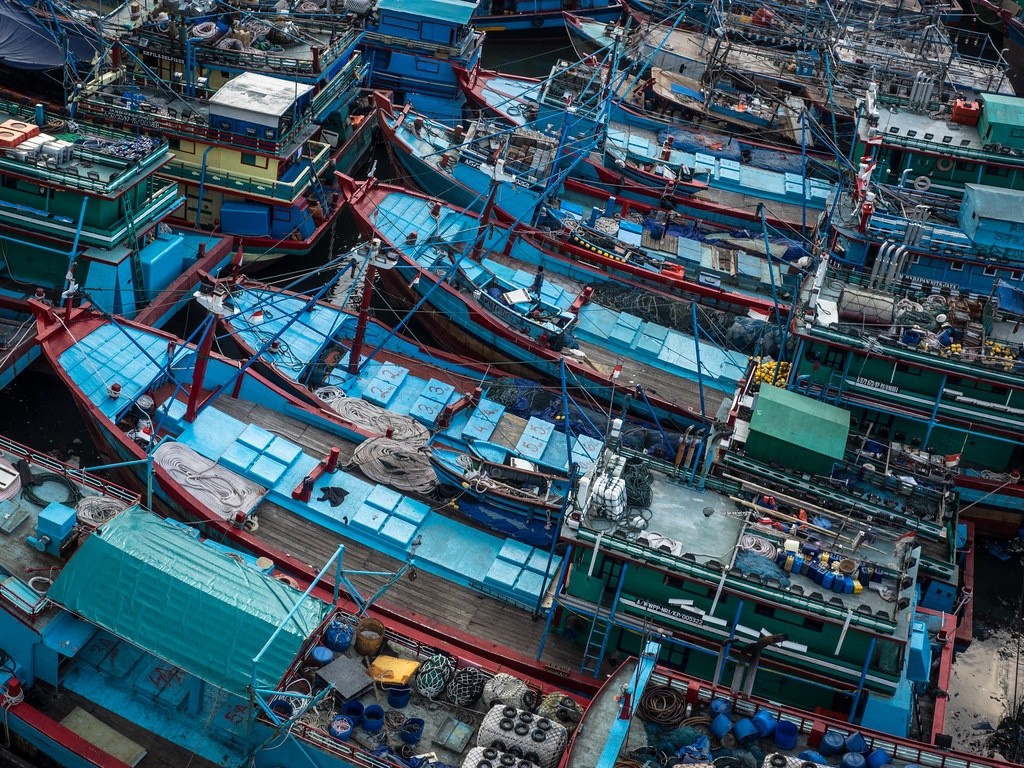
[853,579,863,594]
[881,578,897,590]
[844,577,854,594]
[313,654,374,710]
[515,302,534,313]
[38,500,78,537]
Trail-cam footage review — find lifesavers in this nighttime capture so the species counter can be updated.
[531,730,546,742]
[801,762,818,768]
[661,195,677,209]
[560,697,576,707]
[483,748,498,759]
[490,740,506,752]
[537,718,551,731]
[770,754,787,767]
[497,765,511,768]
[524,752,540,764]
[500,718,514,730]
[914,176,931,190]
[518,760,532,768]
[508,747,522,759]
[477,760,492,768]
[519,712,534,723]
[937,157,953,171]
[556,708,572,722]
[522,691,535,706]
[500,753,515,766]
[514,723,530,735]
[503,706,517,718]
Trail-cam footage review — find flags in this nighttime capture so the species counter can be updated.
[945,453,962,468]
[867,135,884,145]
[896,530,917,543]
[232,241,244,272]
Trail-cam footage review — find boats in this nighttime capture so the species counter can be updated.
[0,1,1024,763]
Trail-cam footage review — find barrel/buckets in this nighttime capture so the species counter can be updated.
[866,747,892,768]
[751,710,777,736]
[845,731,870,755]
[709,714,734,737]
[799,750,828,765]
[363,704,384,732]
[776,721,798,749]
[342,701,364,726]
[818,732,845,757]
[733,719,759,741]
[778,544,884,594]
[706,697,732,720]
[903,330,920,345]
[388,684,411,709]
[840,752,866,768]
[402,718,425,744]
[939,331,951,347]
